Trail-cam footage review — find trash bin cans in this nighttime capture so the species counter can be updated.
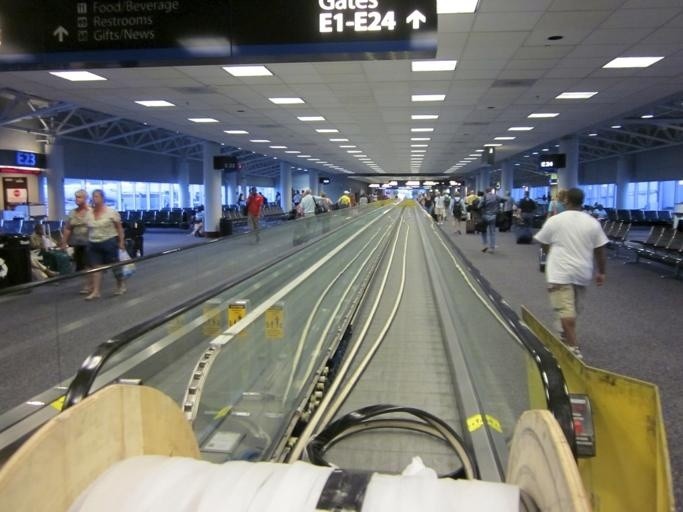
[220,218,232,236]
[0,233,32,296]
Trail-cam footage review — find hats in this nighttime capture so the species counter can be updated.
[344,191,350,194]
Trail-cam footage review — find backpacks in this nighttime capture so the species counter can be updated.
[453,197,464,218]
[443,194,450,207]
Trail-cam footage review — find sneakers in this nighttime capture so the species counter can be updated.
[559,329,568,342]
[80,286,127,300]
[570,346,583,359]
[481,245,494,254]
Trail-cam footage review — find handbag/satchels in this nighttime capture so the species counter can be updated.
[315,204,328,214]
[474,218,487,233]
[243,206,247,216]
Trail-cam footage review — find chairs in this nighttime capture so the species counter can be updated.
[1,201,283,249]
[532,203,683,278]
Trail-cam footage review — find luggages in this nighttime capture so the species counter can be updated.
[516,226,532,243]
[42,220,70,276]
[220,205,232,235]
[467,220,475,233]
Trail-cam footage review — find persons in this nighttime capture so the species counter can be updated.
[186,187,377,241]
[29,187,125,301]
[417,186,610,359]
